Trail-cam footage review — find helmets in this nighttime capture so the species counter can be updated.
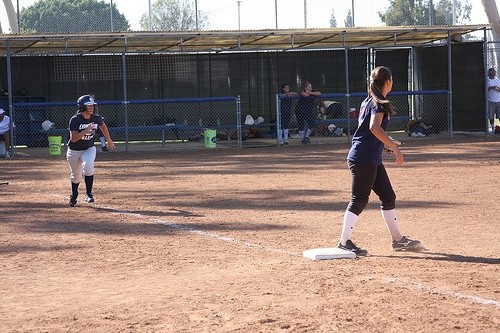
[77,95,98,108]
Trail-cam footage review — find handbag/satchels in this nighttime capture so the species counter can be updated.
[282,123,344,138]
[407,126,428,138]
[217,131,229,140]
[248,127,265,138]
[244,114,265,125]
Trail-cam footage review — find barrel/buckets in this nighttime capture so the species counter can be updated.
[48,136,61,156]
[204,129,216,149]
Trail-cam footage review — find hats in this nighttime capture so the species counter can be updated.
[0,108,6,114]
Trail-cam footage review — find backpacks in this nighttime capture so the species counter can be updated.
[230,128,245,141]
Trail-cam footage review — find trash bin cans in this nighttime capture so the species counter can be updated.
[204,130,216,148]
[270,120,282,139]
[48,136,62,155]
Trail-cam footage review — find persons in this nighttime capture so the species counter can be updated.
[317,101,343,119]
[274,83,299,144]
[67,95,116,207]
[294,82,321,144]
[338,67,421,255]
[488,68,500,133]
[0,109,16,159]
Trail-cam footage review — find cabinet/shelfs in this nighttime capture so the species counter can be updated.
[0,96,50,147]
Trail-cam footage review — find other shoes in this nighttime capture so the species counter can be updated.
[5,151,11,158]
[279,139,289,146]
[69,194,77,206]
[84,192,94,203]
[301,137,310,144]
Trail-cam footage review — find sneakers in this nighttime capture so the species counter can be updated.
[391,235,421,251]
[338,240,367,255]
